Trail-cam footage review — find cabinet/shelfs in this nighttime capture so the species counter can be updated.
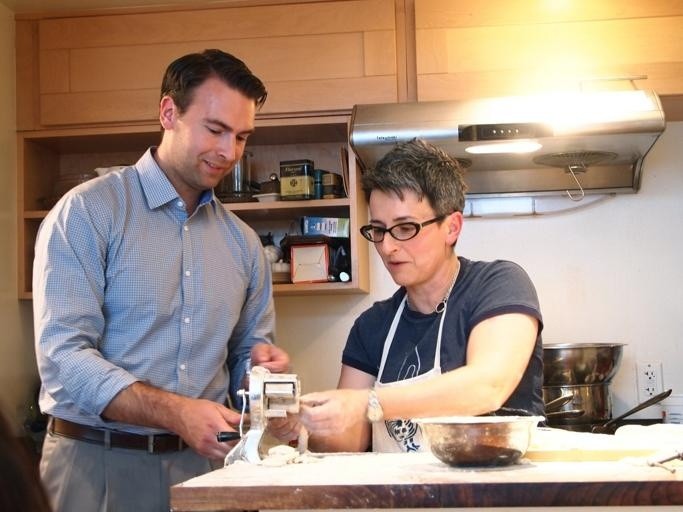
[12,1,683,132]
[13,116,369,301]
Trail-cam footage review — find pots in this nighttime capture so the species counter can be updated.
[537,388,672,434]
[541,383,612,422]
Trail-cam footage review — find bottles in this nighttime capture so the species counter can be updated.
[216,150,254,204]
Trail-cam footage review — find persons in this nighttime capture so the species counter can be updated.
[33,48,290,511]
[266,137,548,454]
[1,404,51,512]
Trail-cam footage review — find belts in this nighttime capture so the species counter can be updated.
[52,417,190,452]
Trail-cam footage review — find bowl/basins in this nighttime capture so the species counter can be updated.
[528,341,630,384]
[410,416,548,467]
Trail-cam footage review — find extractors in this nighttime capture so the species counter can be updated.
[349,87,666,200]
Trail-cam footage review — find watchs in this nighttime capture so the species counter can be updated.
[365,389,384,424]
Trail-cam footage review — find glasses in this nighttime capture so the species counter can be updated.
[360,215,444,243]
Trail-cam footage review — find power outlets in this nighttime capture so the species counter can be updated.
[636,360,665,406]
